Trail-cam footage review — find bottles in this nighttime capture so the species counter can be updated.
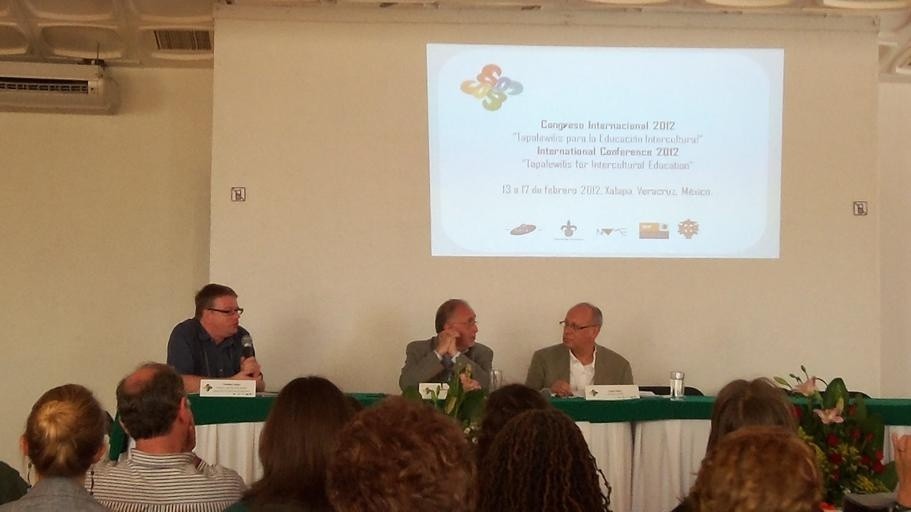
[668,372,684,398]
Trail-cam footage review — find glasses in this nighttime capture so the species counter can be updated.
[560,321,601,333]
[453,319,477,327]
[208,308,243,316]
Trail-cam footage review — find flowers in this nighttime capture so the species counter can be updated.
[774,363,899,511]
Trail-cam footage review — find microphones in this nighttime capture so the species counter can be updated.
[241,335,255,360]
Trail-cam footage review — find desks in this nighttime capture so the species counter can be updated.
[110,385,910,510]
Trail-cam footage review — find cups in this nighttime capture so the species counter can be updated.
[488,369,503,393]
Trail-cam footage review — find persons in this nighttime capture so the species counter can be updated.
[80,362,247,511]
[674,376,911,511]
[399,298,495,399]
[247,374,606,511]
[522,302,636,399]
[2,384,111,510]
[165,283,267,395]
